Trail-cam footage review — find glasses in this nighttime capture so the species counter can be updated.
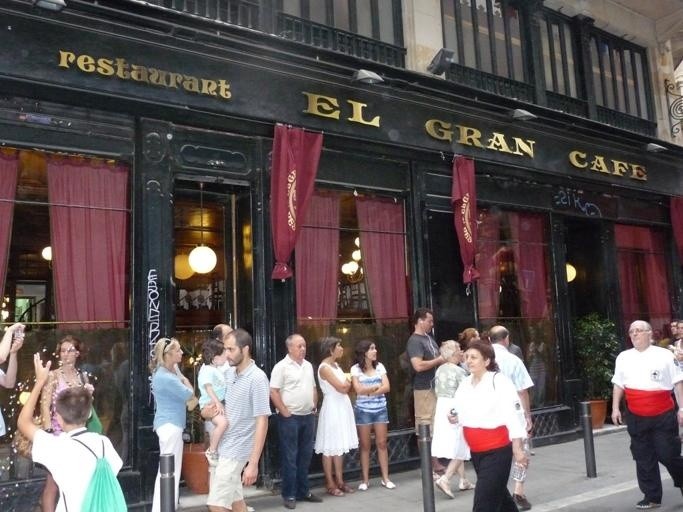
[163,338,171,356]
[628,329,651,334]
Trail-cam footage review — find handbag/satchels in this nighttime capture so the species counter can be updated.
[79,370,103,434]
[511,438,534,482]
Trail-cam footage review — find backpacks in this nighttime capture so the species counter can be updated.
[62,438,127,512]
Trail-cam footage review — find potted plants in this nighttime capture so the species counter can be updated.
[181,343,210,495]
[571,309,620,427]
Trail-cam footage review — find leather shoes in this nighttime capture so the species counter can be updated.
[298,493,322,503]
[285,497,295,508]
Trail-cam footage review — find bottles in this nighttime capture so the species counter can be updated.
[512,443,529,483]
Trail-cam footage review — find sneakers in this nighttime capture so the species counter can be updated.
[635,497,661,509]
[513,494,531,510]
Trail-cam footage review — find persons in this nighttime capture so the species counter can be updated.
[654,329,662,340]
[198,339,227,466]
[150,338,193,512]
[351,340,396,490]
[198,329,271,512]
[407,308,445,481]
[210,324,241,370]
[482,330,493,343]
[611,320,683,509]
[448,340,529,511]
[18,352,127,512]
[0,323,25,438]
[431,340,477,498]
[40,335,103,512]
[490,326,534,508]
[671,321,678,341]
[270,335,323,509]
[508,331,523,359]
[459,328,480,350]
[675,321,683,370]
[401,351,413,426]
[526,323,549,406]
[314,337,359,497]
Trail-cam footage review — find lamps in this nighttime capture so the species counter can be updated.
[189,182,216,274]
[341,237,364,283]
[513,108,537,121]
[42,246,52,270]
[33,1,66,14]
[566,261,576,283]
[647,142,669,153]
[173,208,194,280]
[352,69,385,84]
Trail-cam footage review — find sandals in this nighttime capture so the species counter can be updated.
[458,476,474,490]
[338,484,353,492]
[326,486,343,496]
[358,482,369,490]
[437,476,454,499]
[382,479,395,489]
